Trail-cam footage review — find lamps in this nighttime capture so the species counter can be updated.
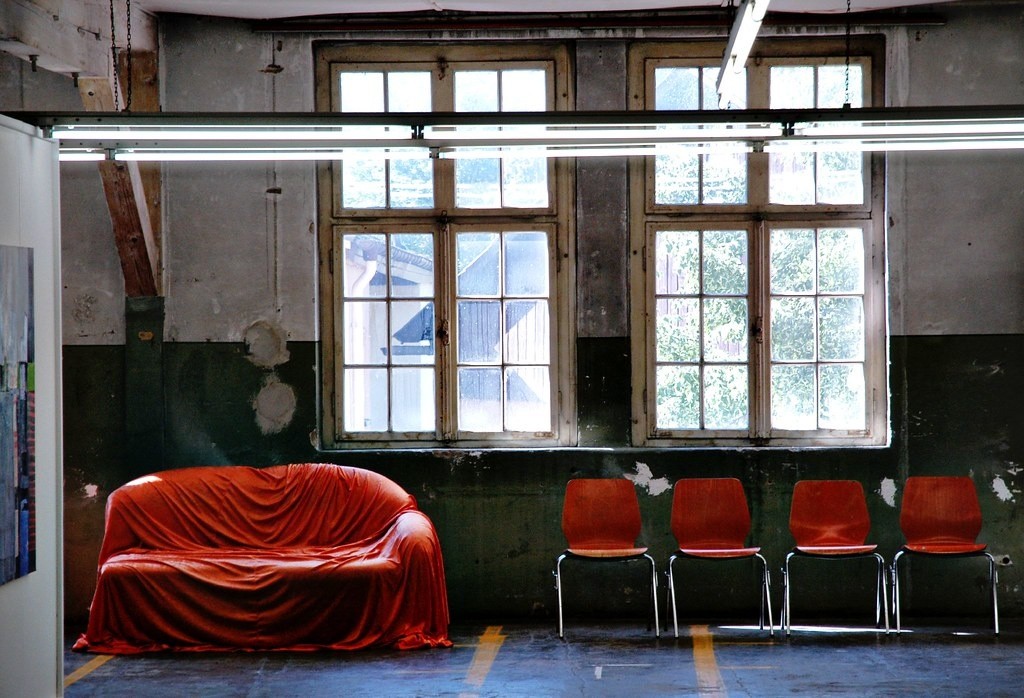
[0,0,1023,164]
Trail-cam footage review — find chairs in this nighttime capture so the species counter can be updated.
[553,477,660,639]
[780,478,891,639]
[891,476,1000,637]
[665,476,776,639]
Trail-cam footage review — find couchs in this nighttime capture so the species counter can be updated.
[70,461,452,654]
